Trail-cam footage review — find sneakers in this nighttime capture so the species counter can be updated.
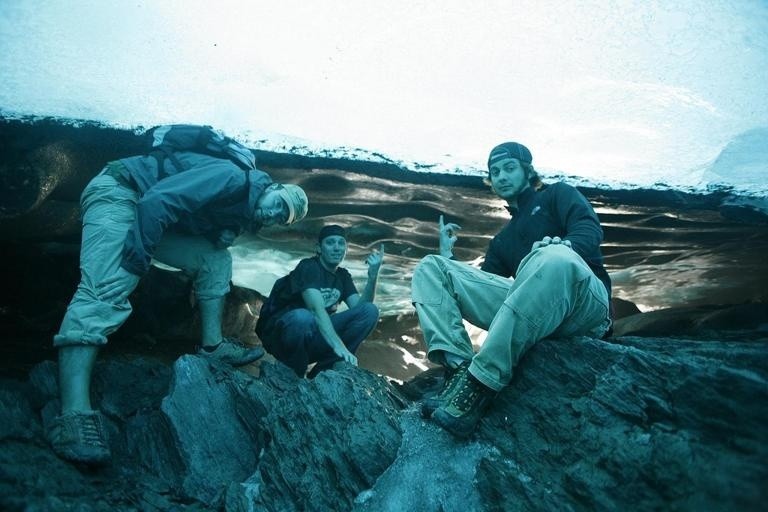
[430,370,496,439]
[43,407,113,466]
[196,336,266,365]
[422,361,472,419]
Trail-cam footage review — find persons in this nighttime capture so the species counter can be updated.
[254,226,385,381]
[39,149,306,465]
[390,142,612,443]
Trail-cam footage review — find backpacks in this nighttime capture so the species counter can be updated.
[143,123,256,172]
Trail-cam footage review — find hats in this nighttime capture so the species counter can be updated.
[487,142,533,169]
[318,225,346,244]
[275,182,309,227]
